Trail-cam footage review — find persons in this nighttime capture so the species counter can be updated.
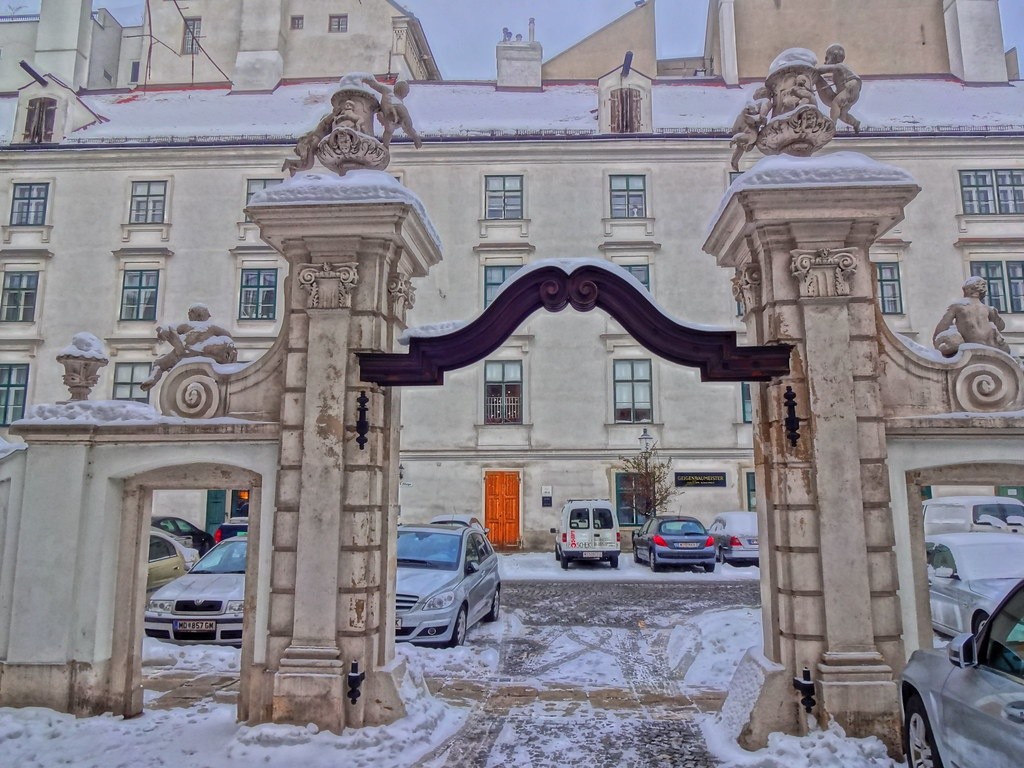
[358,77,426,151]
[729,87,776,172]
[335,100,366,134]
[782,75,812,107]
[814,43,861,138]
[139,302,239,395]
[933,277,1009,355]
[280,111,336,177]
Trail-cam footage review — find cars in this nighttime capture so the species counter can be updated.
[705,511,759,565]
[919,532,1024,643]
[429,514,490,542]
[143,535,247,648]
[396,522,502,648]
[214,502,247,545]
[151,514,214,556]
[631,514,716,573]
[898,580,1024,767]
[148,527,200,592]
[898,573,1024,768]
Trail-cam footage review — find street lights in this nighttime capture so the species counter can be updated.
[638,426,654,521]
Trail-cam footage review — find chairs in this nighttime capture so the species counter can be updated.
[681,524,689,534]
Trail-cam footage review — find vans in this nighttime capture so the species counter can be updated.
[548,498,621,570]
[920,497,1024,535]
